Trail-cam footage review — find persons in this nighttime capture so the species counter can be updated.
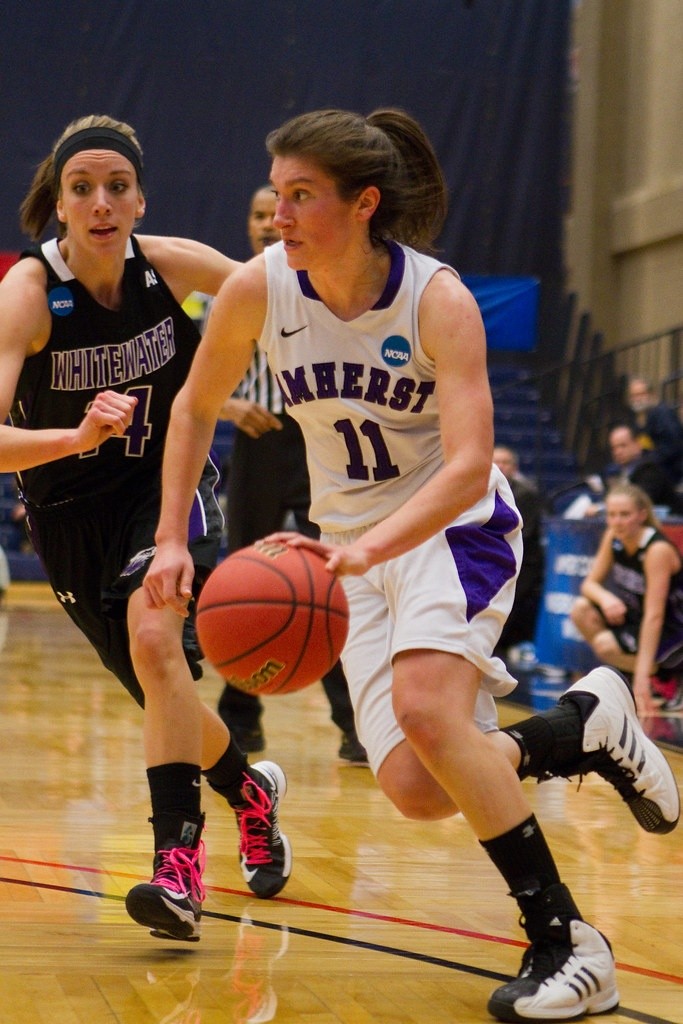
[218,188,367,764]
[492,445,547,658]
[569,375,682,740]
[0,116,291,948]
[142,109,683,1023]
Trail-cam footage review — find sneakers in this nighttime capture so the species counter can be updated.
[559,664,680,835]
[125,812,207,942]
[228,761,292,899]
[487,913,620,1024]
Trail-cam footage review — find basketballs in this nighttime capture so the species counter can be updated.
[194,537,354,701]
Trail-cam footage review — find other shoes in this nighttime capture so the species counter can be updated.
[338,730,369,762]
[644,668,683,748]
[229,723,265,753]
